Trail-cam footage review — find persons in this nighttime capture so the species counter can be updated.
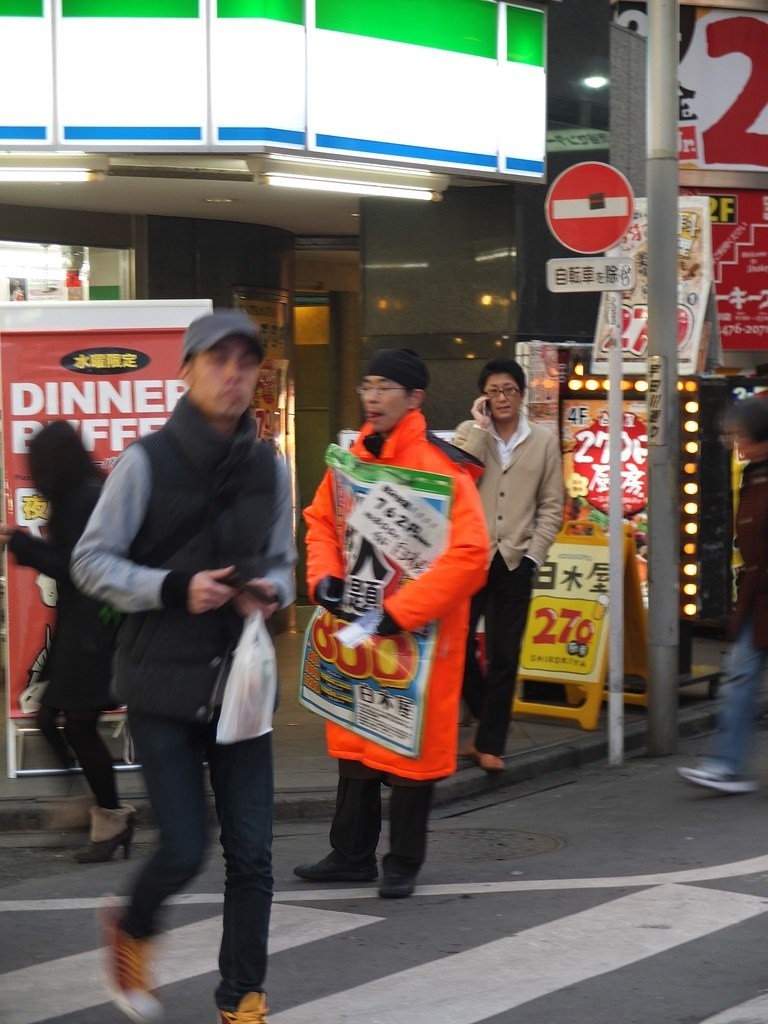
[676,398,768,794]
[70,308,296,1024]
[0,420,137,864]
[448,359,564,770]
[294,346,492,900]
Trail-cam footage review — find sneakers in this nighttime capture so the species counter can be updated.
[379,854,416,896]
[293,849,378,880]
[101,904,165,1024]
[218,991,270,1024]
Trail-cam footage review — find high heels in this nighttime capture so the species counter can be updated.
[73,802,136,864]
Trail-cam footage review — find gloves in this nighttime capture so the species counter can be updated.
[314,575,347,614]
[359,605,402,637]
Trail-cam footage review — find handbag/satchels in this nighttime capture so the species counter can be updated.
[215,610,277,745]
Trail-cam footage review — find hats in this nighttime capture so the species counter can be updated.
[362,347,430,392]
[183,311,267,369]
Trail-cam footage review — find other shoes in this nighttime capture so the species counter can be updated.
[675,759,760,793]
[466,734,508,771]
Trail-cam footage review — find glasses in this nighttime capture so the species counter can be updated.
[356,383,410,400]
[480,387,524,397]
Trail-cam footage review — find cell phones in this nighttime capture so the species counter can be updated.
[483,401,489,417]
